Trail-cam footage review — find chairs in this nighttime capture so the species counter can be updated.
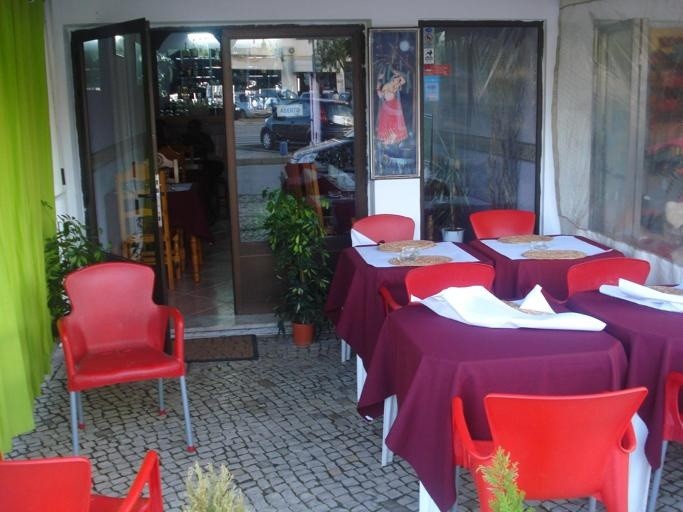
[285,160,334,234]
[342,207,683,512]
[57,261,195,456]
[120,143,228,292]
[0,448,163,512]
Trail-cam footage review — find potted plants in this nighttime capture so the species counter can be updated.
[260,185,337,349]
[425,133,471,242]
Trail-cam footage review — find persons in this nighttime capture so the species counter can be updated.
[159,86,218,161]
[376,72,408,150]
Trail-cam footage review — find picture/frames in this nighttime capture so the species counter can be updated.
[367,25,423,180]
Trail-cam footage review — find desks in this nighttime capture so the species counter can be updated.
[469,235,627,298]
[342,241,496,362]
[357,295,629,512]
[568,283,682,512]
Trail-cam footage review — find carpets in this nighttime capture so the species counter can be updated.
[173,333,259,362]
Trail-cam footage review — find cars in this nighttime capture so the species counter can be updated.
[260,96,356,149]
[285,126,415,178]
[169,85,352,117]
